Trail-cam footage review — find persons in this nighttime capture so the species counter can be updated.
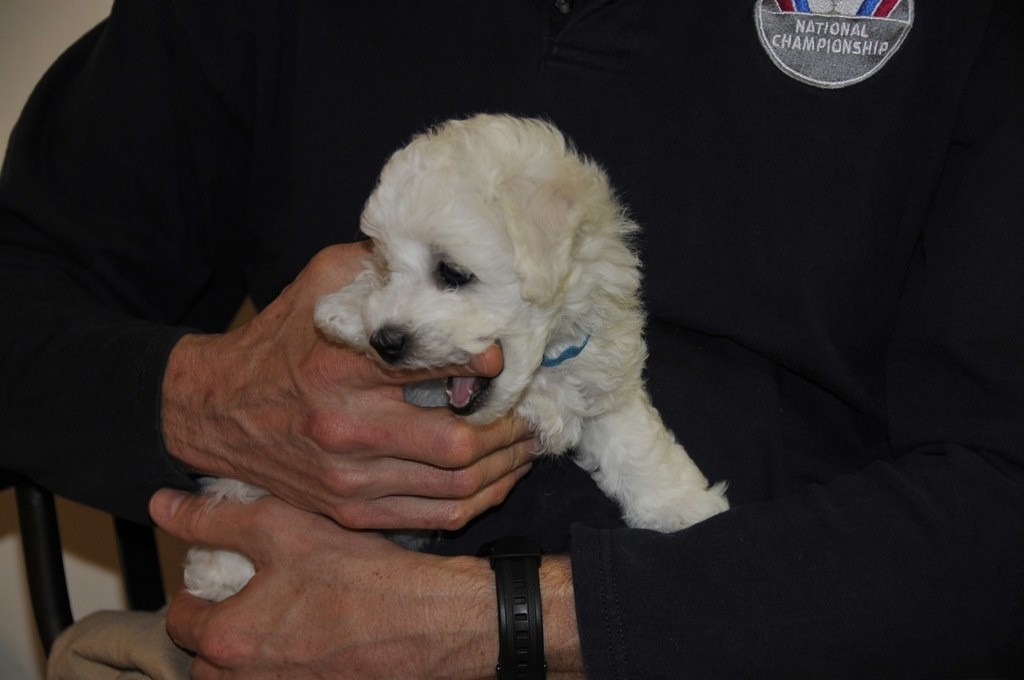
[0,0,1023,680]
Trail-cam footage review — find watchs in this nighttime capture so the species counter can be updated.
[479,538,553,680]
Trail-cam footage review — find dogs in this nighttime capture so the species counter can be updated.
[182,111,730,602]
